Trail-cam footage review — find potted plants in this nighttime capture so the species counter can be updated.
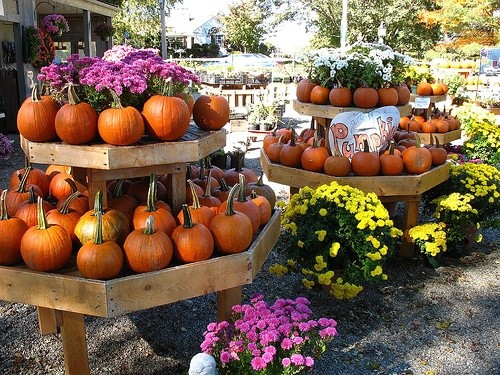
[186,43,220,58]
[443,73,467,105]
[228,146,247,169]
[486,98,500,115]
[247,104,259,130]
[259,120,268,131]
[209,148,228,169]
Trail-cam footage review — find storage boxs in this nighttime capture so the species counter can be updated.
[221,84,243,90]
[246,84,266,89]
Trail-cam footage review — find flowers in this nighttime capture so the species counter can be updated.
[19,14,500,375]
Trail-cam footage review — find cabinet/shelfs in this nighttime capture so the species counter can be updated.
[260,101,450,256]
[408,93,460,145]
[0,121,280,375]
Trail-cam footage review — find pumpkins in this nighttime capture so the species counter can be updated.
[16,77,230,146]
[399,104,461,134]
[296,76,448,108]
[0,152,276,279]
[264,120,448,177]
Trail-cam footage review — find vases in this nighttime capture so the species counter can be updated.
[463,226,477,251]
[51,28,63,36]
[322,270,345,296]
[441,248,464,265]
[100,36,109,41]
[422,252,441,269]
